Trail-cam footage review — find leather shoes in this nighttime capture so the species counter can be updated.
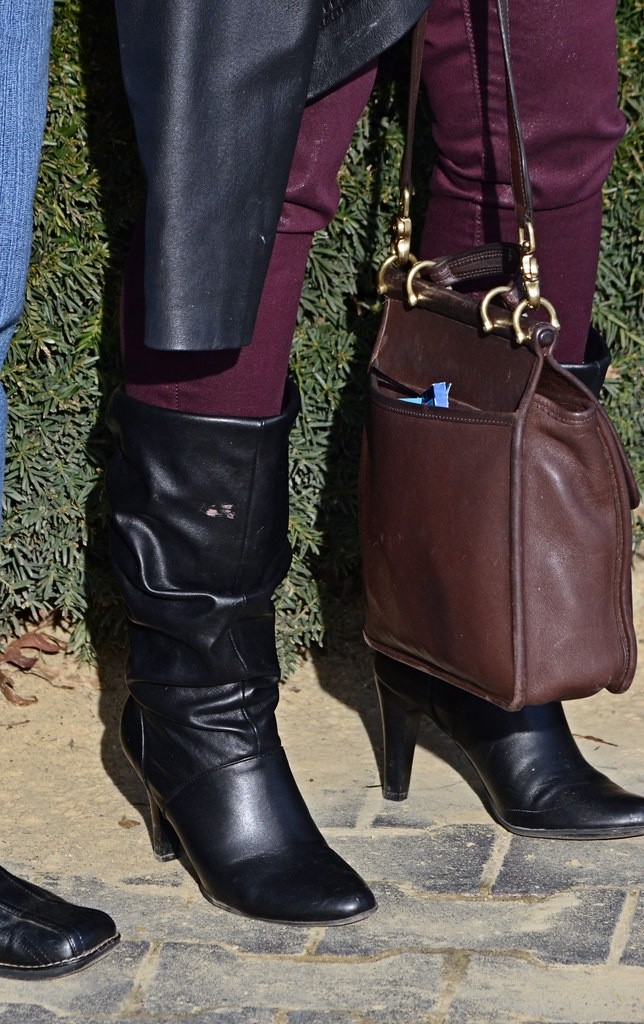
[1,864,123,984]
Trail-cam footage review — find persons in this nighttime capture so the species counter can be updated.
[81,1,644,921]
[1,1,122,985]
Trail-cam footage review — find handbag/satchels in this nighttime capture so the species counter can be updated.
[360,0,643,715]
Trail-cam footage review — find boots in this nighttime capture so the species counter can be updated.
[373,325,644,842]
[100,366,380,931]
